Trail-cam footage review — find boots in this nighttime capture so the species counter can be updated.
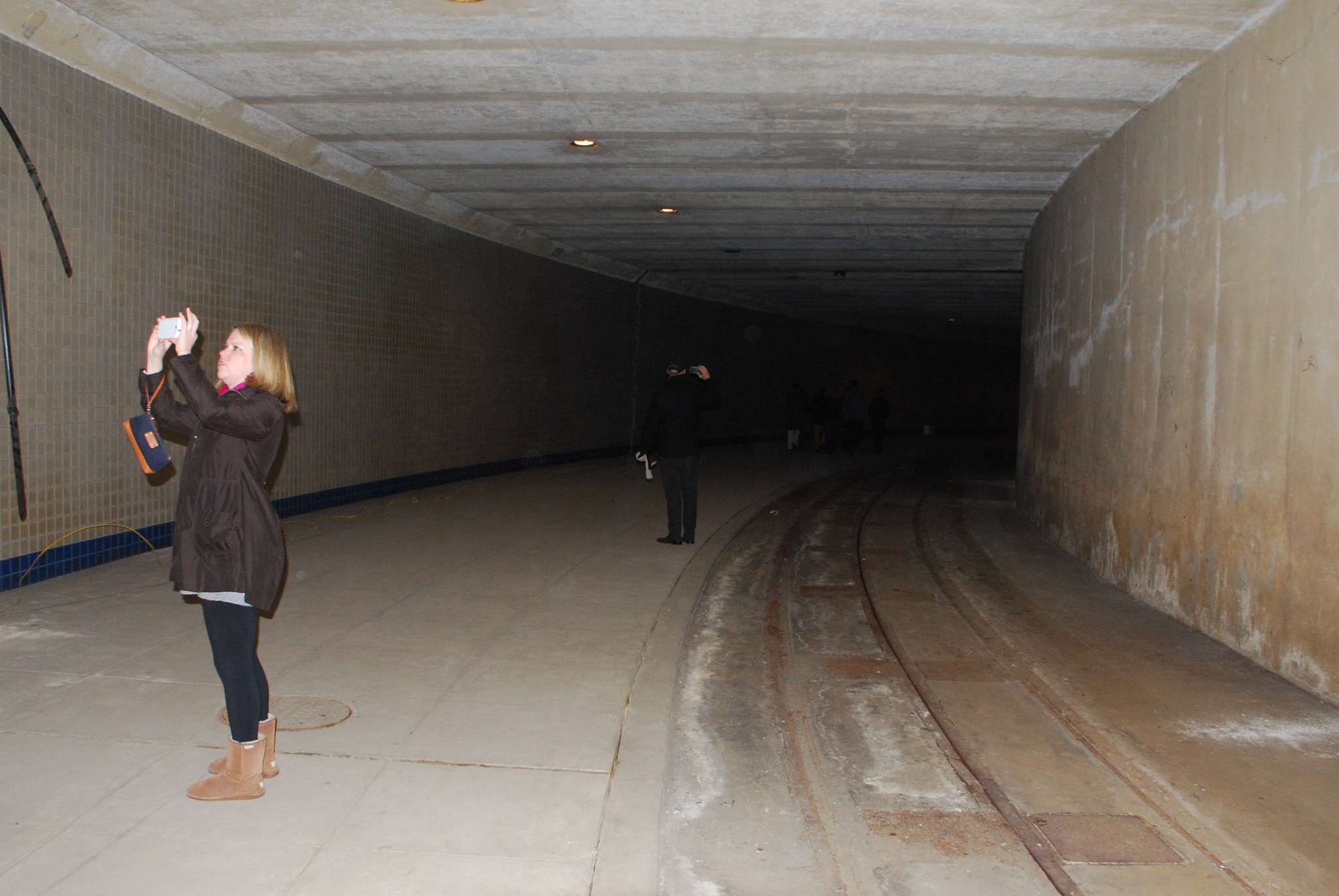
[186,730,267,800]
[208,712,279,778]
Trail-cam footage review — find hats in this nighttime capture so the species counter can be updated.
[668,357,687,374]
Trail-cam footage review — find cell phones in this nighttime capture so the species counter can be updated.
[157,317,181,339]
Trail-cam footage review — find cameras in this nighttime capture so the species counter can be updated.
[690,367,702,373]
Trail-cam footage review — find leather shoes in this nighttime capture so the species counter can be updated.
[657,535,682,545]
[682,536,694,544]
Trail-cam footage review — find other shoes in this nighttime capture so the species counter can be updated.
[788,444,858,456]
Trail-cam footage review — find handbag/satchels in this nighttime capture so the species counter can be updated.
[123,374,171,474]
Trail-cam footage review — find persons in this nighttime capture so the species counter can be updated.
[640,356,721,545]
[922,391,937,439]
[140,307,298,801]
[785,378,865,454]
[868,387,890,455]
